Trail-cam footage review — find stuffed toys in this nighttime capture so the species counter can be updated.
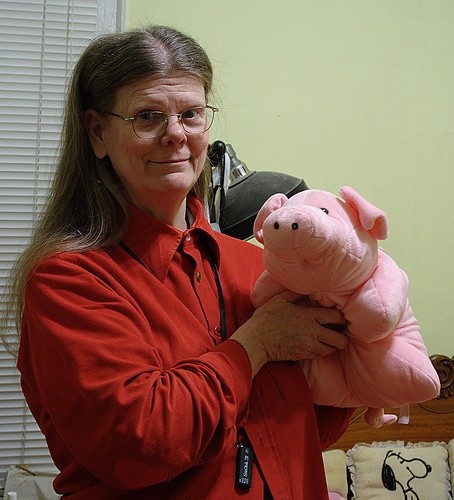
[249,186,442,429]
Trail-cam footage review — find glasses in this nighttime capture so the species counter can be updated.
[95,104,219,139]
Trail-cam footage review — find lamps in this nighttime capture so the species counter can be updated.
[209,138,310,242]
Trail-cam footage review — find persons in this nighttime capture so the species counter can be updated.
[1,24,349,500]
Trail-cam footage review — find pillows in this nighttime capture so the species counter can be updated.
[345,441,453,500]
